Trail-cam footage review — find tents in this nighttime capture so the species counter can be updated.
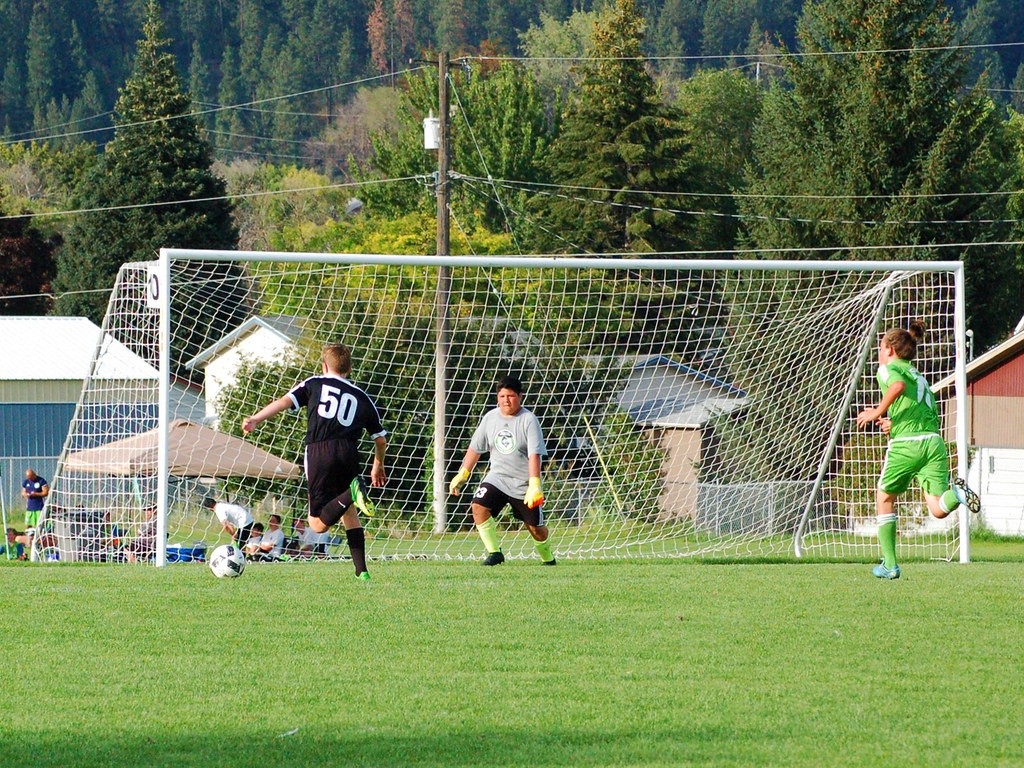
[54,421,302,559]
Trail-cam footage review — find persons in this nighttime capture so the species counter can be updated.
[247,514,285,564]
[118,503,157,556]
[242,343,387,580]
[0,527,24,560]
[293,517,332,560]
[856,320,981,580]
[22,468,49,530]
[24,525,36,546]
[449,376,556,566]
[203,497,254,558]
[246,522,264,559]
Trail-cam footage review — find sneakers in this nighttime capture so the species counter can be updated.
[354,571,370,581]
[350,476,375,516]
[481,547,505,566]
[953,477,981,513]
[872,561,900,579]
[542,558,557,565]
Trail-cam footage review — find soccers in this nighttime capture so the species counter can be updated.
[208,544,246,581]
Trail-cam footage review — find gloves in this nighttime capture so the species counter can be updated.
[524,477,544,509]
[450,466,470,495]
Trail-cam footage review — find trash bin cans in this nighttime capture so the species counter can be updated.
[50,510,103,563]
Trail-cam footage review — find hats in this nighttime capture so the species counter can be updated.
[142,503,157,510]
